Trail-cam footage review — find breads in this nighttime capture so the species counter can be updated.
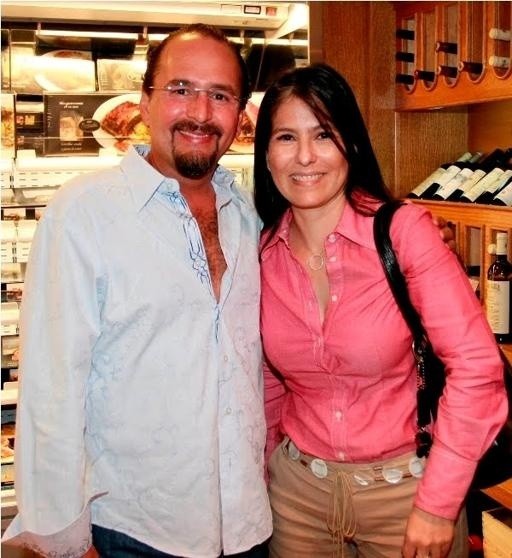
[99,101,142,141]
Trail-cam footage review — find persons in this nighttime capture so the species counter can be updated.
[2,26,278,558]
[249,61,510,557]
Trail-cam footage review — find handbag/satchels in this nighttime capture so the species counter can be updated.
[418,346,512,492]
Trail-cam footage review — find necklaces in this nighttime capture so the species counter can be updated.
[307,249,324,270]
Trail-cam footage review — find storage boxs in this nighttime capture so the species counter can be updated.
[481,506,512,558]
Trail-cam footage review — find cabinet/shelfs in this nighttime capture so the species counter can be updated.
[1,2,309,534]
[391,0,512,558]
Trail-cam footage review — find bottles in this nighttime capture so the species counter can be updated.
[394,27,512,342]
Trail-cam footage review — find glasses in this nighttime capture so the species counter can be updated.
[147,82,244,109]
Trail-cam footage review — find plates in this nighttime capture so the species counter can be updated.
[227,100,259,154]
[34,47,95,93]
[91,91,146,156]
